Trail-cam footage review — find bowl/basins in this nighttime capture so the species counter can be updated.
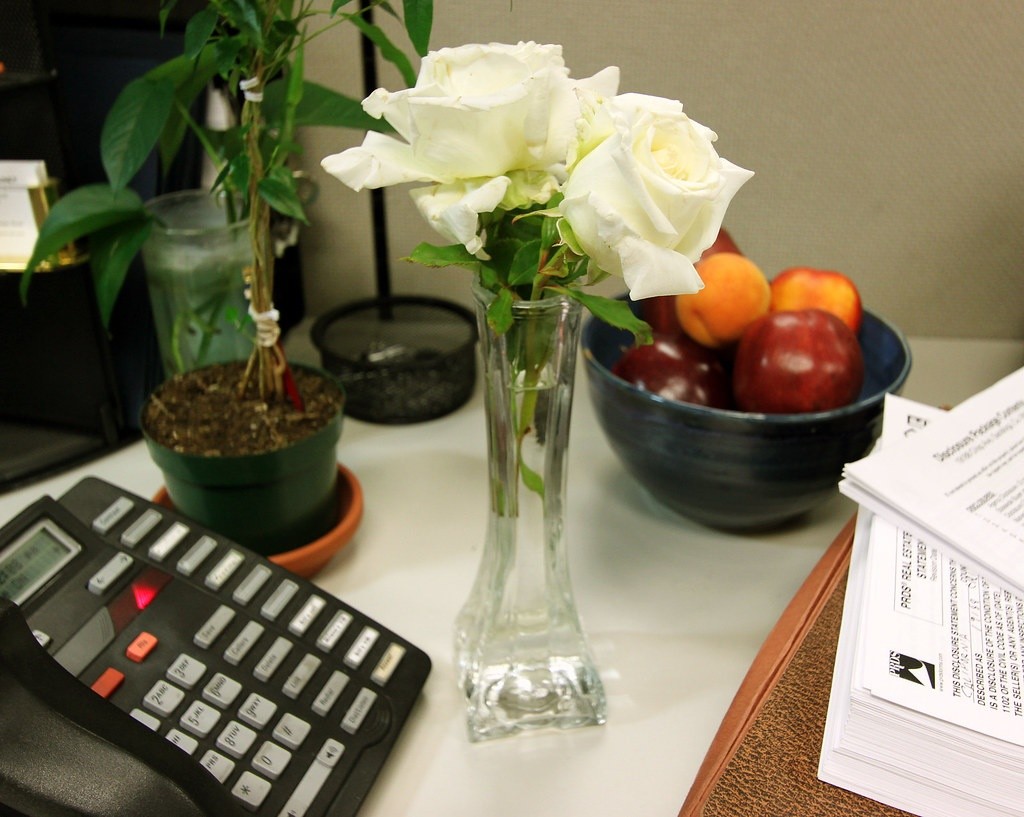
[581,291,912,537]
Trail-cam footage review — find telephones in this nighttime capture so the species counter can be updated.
[0,475,433,817]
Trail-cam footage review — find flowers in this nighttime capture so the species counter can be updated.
[320,36,754,514]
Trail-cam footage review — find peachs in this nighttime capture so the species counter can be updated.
[671,250,770,346]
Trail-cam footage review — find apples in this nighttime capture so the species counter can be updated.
[606,226,862,414]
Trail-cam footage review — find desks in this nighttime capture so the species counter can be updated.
[0,338,1024,817]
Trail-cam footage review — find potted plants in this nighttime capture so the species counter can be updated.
[33,1,438,578]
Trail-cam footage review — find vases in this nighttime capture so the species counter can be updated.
[452,272,609,746]
[130,188,266,379]
